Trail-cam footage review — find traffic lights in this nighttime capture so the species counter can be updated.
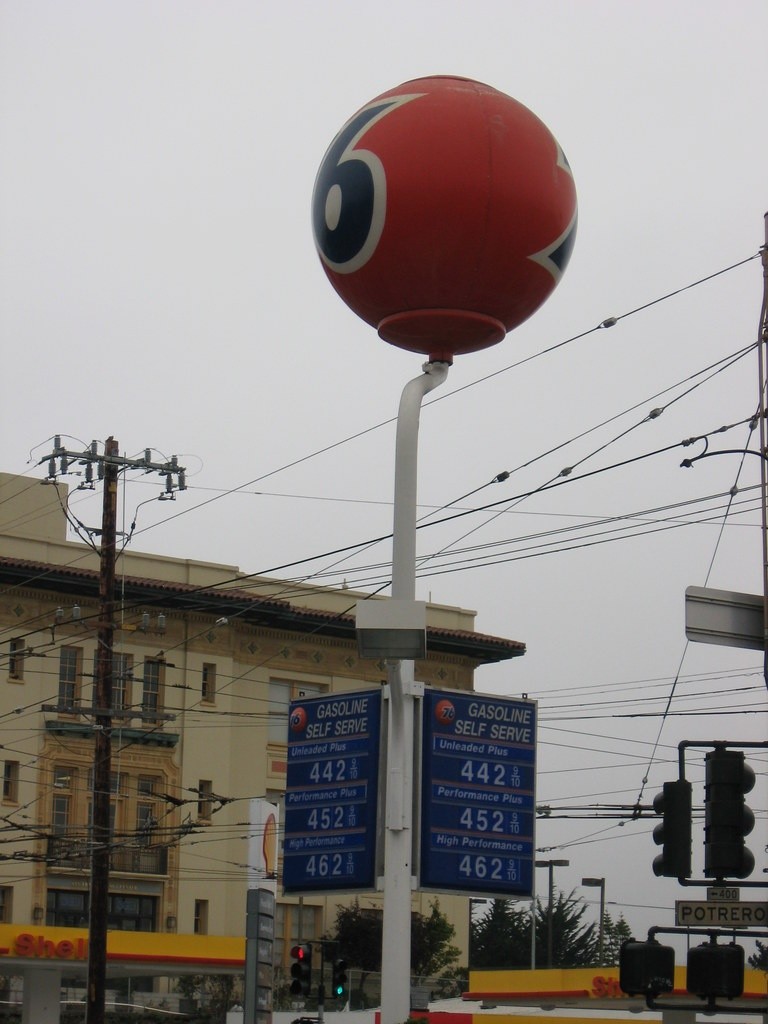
[334,959,347,998]
[652,779,693,877]
[290,944,309,996]
